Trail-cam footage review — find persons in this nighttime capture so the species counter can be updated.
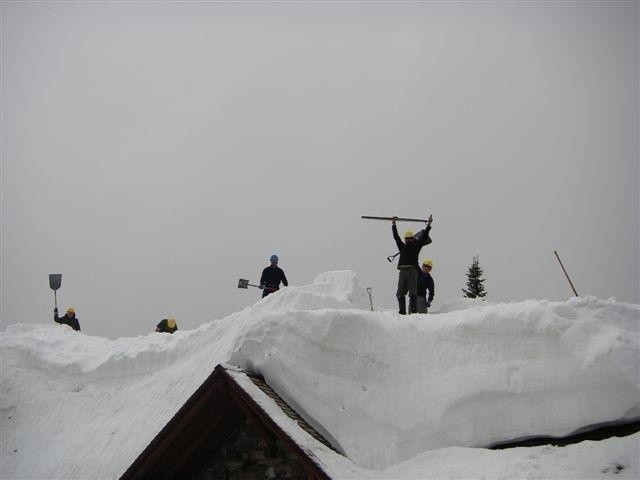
[259,254,288,299]
[54,307,80,331]
[392,214,434,315]
[413,259,434,313]
[156,318,178,334]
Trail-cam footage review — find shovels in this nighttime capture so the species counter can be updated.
[49,274,62,308]
[238,279,278,292]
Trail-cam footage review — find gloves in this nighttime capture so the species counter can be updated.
[392,216,398,224]
[54,307,58,313]
[428,214,433,226]
[426,300,431,307]
[259,285,264,289]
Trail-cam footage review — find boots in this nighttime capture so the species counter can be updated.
[399,295,417,315]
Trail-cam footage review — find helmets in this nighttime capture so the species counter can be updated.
[67,307,75,313]
[404,231,413,238]
[167,320,176,328]
[270,255,279,262]
[423,258,433,267]
